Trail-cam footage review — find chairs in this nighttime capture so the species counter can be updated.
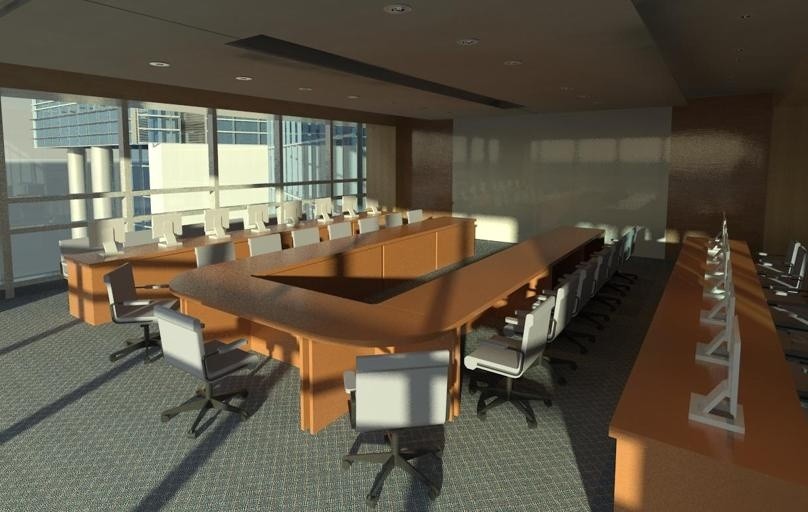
[380,223,641,429]
[102,262,179,365]
[342,351,452,504]
[154,309,250,439]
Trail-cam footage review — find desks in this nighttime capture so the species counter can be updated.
[60,206,476,324]
[607,221,808,511]
[170,266,448,437]
